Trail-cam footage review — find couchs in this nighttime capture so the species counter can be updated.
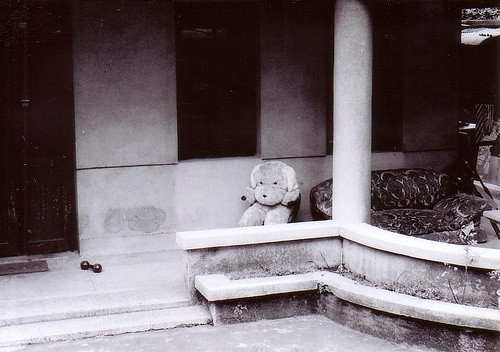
[310,168,491,245]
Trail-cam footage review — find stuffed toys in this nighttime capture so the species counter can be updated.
[237,160,302,226]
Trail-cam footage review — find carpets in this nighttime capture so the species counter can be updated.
[0,260,48,275]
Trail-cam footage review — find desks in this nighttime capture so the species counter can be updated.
[484,210,500,240]
